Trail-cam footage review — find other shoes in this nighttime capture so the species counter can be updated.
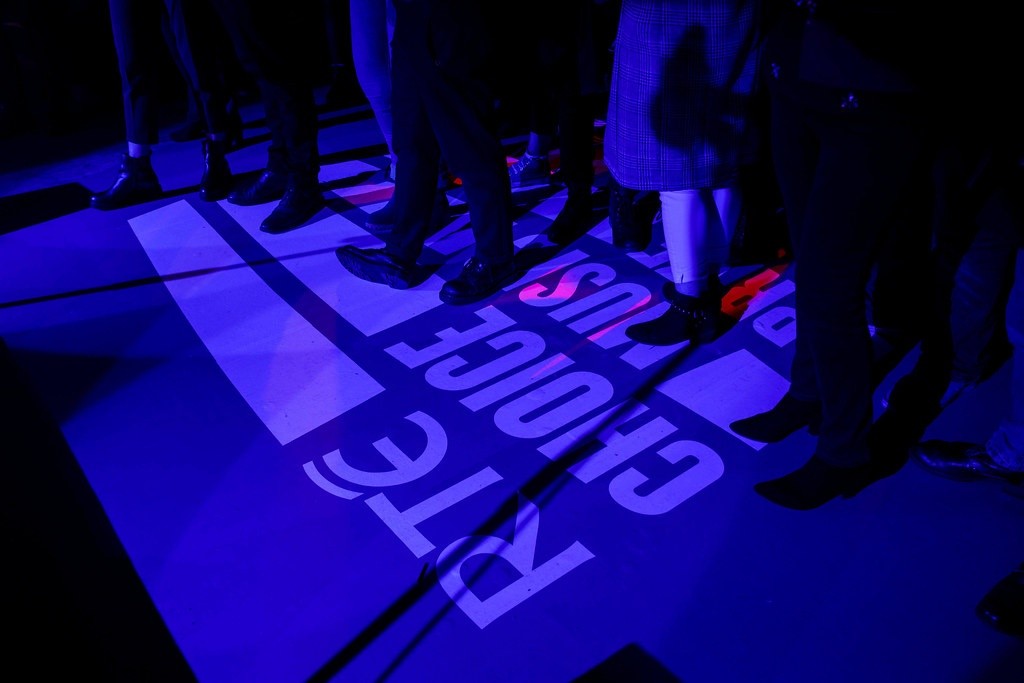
[754,455,872,512]
[508,152,551,194]
[548,200,591,244]
[634,203,653,251]
[975,558,1024,641]
[608,197,629,248]
[201,115,245,154]
[226,169,284,206]
[729,391,822,442]
[364,189,450,232]
[260,179,327,233]
[170,114,204,141]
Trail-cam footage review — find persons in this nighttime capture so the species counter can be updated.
[506,0,1024,641]
[90,0,324,235]
[334,0,523,305]
[349,0,449,229]
[602,0,770,346]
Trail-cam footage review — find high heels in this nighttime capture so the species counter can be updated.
[664,265,746,340]
[625,290,707,349]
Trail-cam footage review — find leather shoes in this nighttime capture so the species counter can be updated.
[199,147,238,201]
[438,256,522,304]
[334,244,419,291]
[89,153,162,209]
